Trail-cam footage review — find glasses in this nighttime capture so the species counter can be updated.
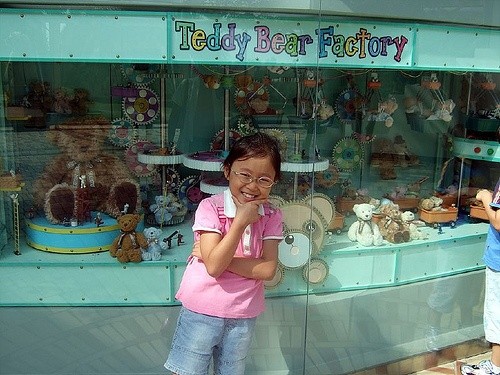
[229,168,278,188]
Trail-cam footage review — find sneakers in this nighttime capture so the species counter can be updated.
[460,360,500,375]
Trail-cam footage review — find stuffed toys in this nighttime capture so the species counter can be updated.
[1,62,500,266]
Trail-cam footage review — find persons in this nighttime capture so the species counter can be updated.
[460,186,500,375]
[160,132,287,375]
